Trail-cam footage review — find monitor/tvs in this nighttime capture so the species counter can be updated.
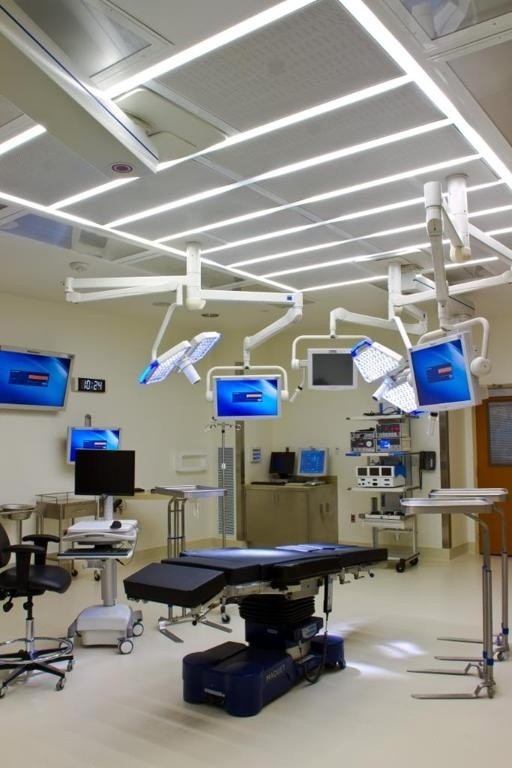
[296,447,328,484]
[74,448,135,497]
[213,374,282,419]
[269,451,295,479]
[307,348,357,390]
[0,345,75,411]
[408,331,483,410]
[67,425,120,466]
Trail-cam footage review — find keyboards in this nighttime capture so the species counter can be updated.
[57,548,129,556]
[251,481,285,485]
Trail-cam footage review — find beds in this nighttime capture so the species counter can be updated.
[123,538,391,720]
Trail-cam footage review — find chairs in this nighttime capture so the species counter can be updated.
[0,523,73,699]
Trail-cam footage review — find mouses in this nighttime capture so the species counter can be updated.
[110,520,121,529]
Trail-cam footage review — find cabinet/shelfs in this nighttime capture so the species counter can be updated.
[345,414,424,571]
[242,476,339,547]
